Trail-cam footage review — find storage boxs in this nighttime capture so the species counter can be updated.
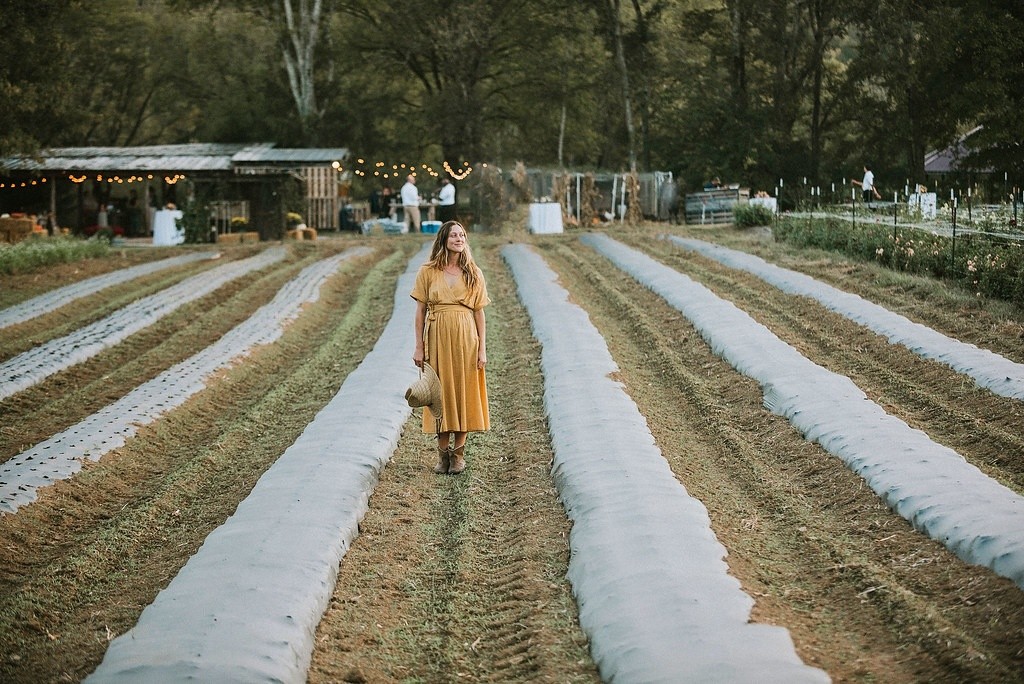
[7,228,49,243]
[0,217,34,233]
[422,221,443,233]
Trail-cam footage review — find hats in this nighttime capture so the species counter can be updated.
[405,358,444,419]
[437,176,444,183]
[406,174,414,180]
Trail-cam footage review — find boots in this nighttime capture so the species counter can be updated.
[448,444,465,474]
[433,446,450,474]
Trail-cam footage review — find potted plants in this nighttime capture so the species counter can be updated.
[286,212,307,230]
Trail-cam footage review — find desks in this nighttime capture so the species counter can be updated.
[389,201,440,223]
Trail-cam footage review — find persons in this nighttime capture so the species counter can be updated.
[430,178,456,223]
[598,207,609,222]
[410,221,491,474]
[401,175,422,232]
[862,165,875,202]
[702,177,720,192]
